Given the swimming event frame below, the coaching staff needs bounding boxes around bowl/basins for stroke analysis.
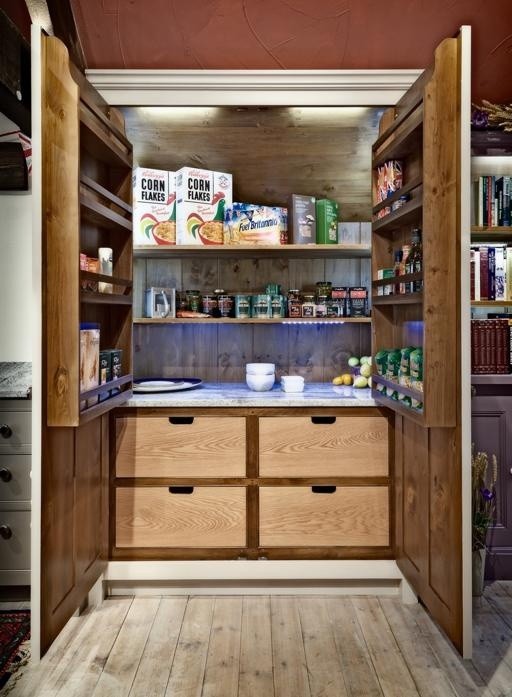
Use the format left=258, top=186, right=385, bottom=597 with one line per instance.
left=281, top=374, right=305, bottom=393
left=244, top=362, right=276, bottom=391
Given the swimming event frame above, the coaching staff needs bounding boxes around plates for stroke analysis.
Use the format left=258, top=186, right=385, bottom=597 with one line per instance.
left=132, top=376, right=204, bottom=393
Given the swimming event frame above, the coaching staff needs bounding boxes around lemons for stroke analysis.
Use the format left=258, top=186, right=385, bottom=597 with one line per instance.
left=334, top=375, right=352, bottom=386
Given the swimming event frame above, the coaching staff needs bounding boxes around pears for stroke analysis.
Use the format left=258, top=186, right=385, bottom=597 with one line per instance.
left=348, top=356, right=371, bottom=388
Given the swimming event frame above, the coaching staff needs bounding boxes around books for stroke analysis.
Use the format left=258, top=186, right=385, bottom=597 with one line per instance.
left=470, top=169, right=511, bottom=378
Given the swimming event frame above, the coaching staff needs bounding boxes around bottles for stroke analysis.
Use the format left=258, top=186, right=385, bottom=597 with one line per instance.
left=391, top=227, right=423, bottom=294
left=288, top=281, right=367, bottom=317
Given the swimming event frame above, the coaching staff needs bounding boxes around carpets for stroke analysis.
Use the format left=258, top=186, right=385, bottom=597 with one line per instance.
left=0, top=608, right=36, bottom=697
